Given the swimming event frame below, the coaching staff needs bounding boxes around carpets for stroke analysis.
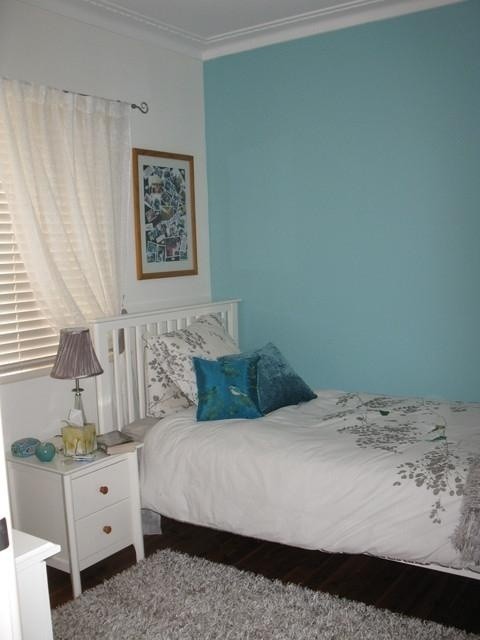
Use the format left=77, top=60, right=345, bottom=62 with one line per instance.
left=53, top=548, right=480, bottom=640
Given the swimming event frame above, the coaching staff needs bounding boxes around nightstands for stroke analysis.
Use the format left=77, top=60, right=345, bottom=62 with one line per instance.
left=13, top=441, right=145, bottom=598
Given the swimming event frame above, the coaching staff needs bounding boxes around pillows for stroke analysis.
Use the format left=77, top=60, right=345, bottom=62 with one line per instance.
left=137, top=314, right=316, bottom=425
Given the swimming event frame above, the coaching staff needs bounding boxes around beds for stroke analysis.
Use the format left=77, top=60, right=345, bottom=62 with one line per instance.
left=78, top=297, right=480, bottom=580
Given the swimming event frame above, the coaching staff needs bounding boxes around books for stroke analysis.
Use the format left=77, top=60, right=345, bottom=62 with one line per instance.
left=96, top=429, right=136, bottom=456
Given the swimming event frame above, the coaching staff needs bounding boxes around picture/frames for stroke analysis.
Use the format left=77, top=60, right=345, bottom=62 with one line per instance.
left=130, top=147, right=199, bottom=282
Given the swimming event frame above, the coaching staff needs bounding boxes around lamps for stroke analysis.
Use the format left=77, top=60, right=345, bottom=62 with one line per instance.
left=52, top=327, right=105, bottom=426
